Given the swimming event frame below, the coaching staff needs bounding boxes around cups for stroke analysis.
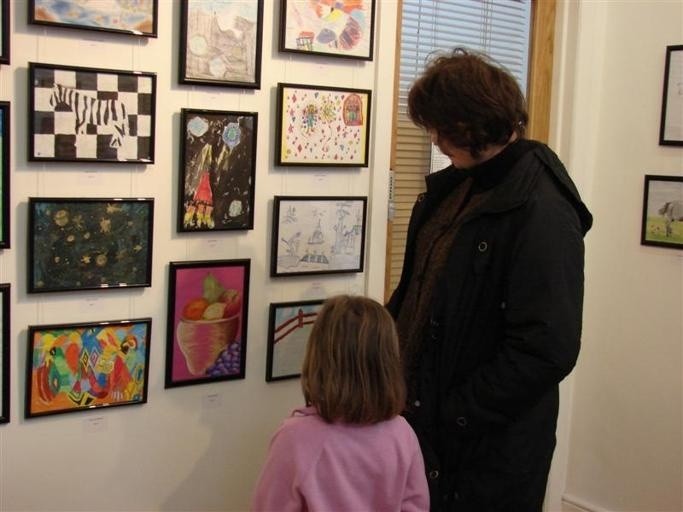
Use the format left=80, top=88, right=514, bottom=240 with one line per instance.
left=177, top=313, right=240, bottom=376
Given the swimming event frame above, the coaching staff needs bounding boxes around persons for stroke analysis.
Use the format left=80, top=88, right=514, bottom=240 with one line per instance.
left=384, top=46, right=593, bottom=512
left=247, top=294, right=432, bottom=512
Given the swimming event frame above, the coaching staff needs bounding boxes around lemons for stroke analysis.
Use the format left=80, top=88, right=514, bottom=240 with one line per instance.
left=201, top=303, right=226, bottom=320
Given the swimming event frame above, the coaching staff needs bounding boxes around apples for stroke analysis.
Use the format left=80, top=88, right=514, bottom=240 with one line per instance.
left=220, top=290, right=240, bottom=317
left=182, top=299, right=208, bottom=321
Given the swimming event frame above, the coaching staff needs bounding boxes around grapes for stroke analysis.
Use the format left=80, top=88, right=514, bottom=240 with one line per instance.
left=206, top=342, right=241, bottom=378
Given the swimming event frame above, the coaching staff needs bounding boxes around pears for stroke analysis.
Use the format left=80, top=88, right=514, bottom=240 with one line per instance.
left=202, top=272, right=220, bottom=302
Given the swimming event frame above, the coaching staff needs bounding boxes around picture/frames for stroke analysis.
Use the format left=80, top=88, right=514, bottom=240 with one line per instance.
left=638, top=44, right=682, bottom=250
